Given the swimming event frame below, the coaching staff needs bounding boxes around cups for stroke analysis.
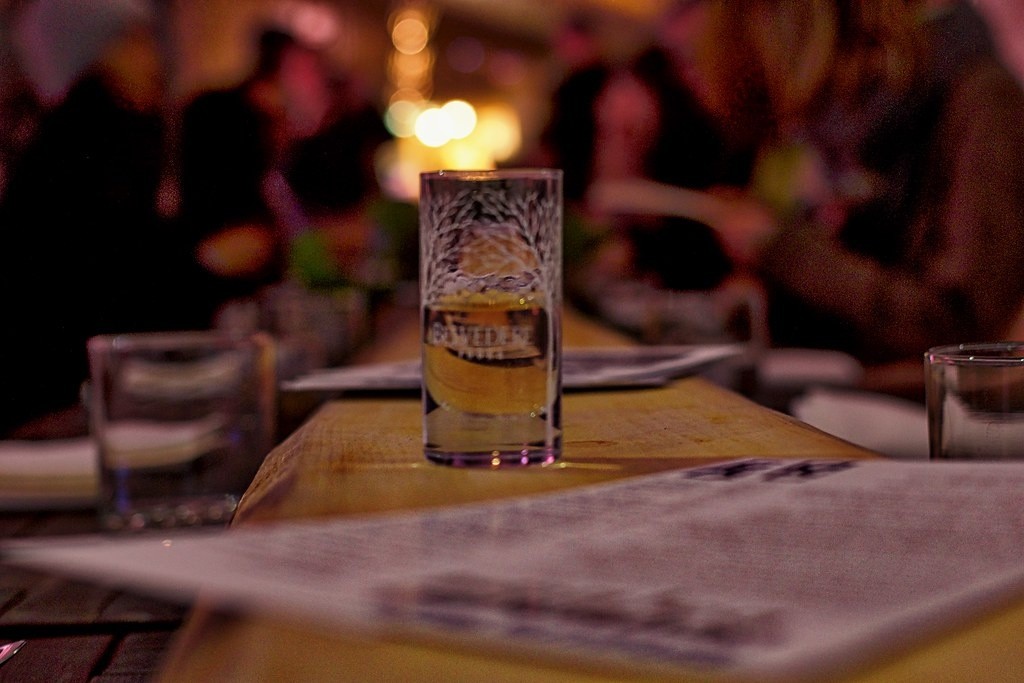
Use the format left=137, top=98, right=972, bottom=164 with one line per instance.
left=419, top=170, right=563, bottom=468
left=89, top=335, right=276, bottom=535
left=924, top=341, right=1024, bottom=461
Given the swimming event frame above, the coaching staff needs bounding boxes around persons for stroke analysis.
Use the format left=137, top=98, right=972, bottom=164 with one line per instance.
left=0, top=1, right=1024, bottom=402
